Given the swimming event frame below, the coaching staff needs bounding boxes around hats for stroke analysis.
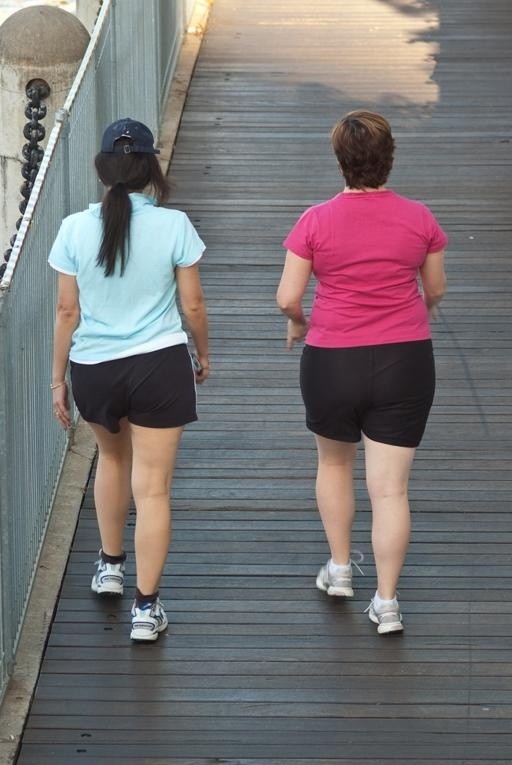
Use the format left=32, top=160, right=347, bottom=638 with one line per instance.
left=101, top=118, right=161, bottom=155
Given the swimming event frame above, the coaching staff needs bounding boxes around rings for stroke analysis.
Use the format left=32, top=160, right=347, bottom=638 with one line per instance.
left=56, top=413, right=59, bottom=416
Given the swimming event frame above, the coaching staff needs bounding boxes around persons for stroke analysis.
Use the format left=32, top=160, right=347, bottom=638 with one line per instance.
left=43, top=117, right=210, bottom=644
left=275, top=108, right=448, bottom=637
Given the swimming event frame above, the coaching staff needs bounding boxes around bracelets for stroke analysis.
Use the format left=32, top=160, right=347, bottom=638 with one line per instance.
left=50, top=380, right=66, bottom=390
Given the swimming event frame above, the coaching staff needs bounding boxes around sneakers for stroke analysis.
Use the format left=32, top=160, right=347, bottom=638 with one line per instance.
left=368, top=591, right=404, bottom=635
left=91, top=548, right=126, bottom=597
left=130, top=599, right=169, bottom=642
left=316, top=558, right=355, bottom=598
